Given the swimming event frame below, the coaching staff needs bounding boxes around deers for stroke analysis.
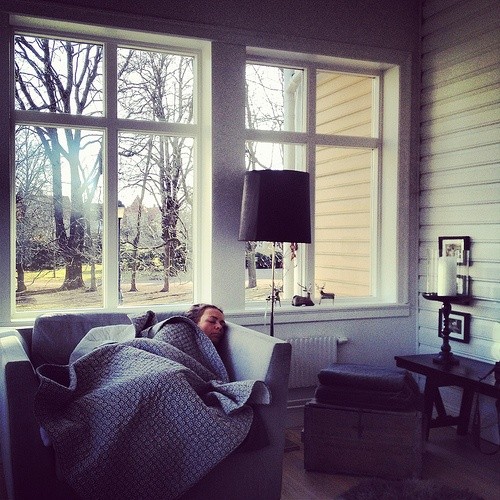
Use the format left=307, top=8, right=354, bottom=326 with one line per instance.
left=265, top=284, right=284, bottom=307
left=291, top=282, right=315, bottom=307
left=316, top=282, right=335, bottom=305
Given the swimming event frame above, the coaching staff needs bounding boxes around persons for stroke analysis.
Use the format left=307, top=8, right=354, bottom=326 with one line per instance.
left=186, top=306, right=226, bottom=347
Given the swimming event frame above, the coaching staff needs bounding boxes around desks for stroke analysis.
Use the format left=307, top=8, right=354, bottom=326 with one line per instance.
left=395, top=354, right=500, bottom=443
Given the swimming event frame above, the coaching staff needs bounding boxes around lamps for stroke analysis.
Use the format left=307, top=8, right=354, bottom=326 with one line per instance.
left=239, top=171, right=312, bottom=452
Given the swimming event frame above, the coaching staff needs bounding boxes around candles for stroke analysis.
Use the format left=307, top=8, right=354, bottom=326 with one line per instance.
left=438, top=257, right=457, bottom=296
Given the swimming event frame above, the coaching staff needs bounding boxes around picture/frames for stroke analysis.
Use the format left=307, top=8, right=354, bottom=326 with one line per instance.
left=439, top=236, right=470, bottom=265
left=455, top=275, right=470, bottom=297
left=438, top=309, right=471, bottom=342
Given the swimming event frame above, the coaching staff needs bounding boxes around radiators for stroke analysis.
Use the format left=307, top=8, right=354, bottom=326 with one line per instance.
left=284, top=335, right=337, bottom=388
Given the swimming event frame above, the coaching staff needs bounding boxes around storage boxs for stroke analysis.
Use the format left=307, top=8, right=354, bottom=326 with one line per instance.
left=302, top=394, right=425, bottom=481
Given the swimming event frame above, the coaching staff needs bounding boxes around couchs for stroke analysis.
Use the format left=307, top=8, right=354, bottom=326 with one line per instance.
left=0, top=311, right=292, bottom=500
